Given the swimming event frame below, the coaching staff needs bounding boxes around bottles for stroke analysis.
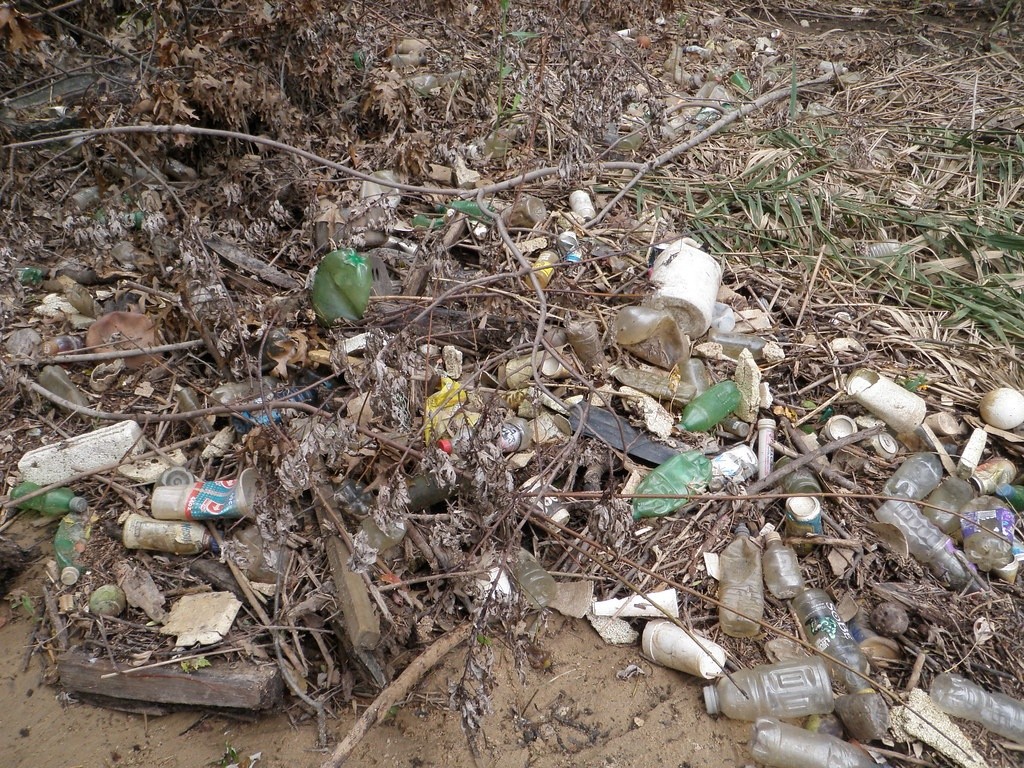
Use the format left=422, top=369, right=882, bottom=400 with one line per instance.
left=868, top=239, right=902, bottom=264
left=9, top=483, right=89, bottom=517
left=13, top=267, right=42, bottom=287
left=122, top=514, right=216, bottom=550
left=178, top=371, right=331, bottom=448
left=411, top=197, right=568, bottom=292
left=334, top=481, right=373, bottom=520
left=351, top=34, right=470, bottom=101
left=311, top=247, right=369, bottom=329
left=606, top=29, right=801, bottom=154
left=54, top=513, right=89, bottom=586
left=647, top=327, right=1024, bottom=767
left=817, top=61, right=850, bottom=74
left=63, top=150, right=203, bottom=327
left=806, top=102, right=841, bottom=123
left=41, top=335, right=84, bottom=355
left=357, top=323, right=607, bottom=610
left=40, top=367, right=89, bottom=416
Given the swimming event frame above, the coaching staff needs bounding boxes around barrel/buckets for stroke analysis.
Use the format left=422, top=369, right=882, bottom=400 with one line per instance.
left=639, top=245, right=723, bottom=341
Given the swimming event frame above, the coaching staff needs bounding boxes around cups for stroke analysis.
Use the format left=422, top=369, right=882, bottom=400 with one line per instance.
left=521, top=476, right=572, bottom=532
left=642, top=618, right=725, bottom=678
left=151, top=467, right=267, bottom=518
